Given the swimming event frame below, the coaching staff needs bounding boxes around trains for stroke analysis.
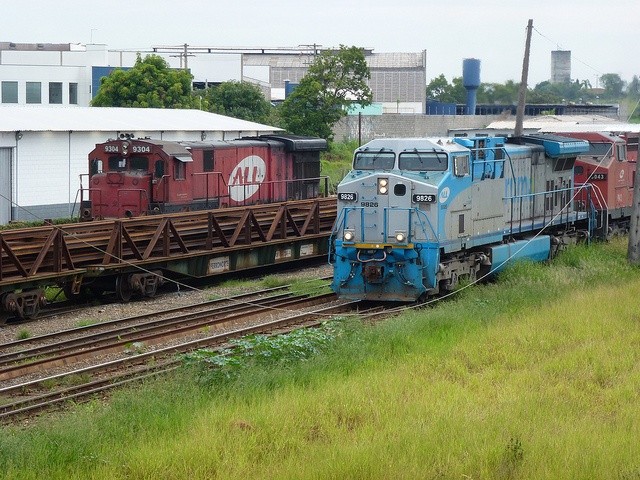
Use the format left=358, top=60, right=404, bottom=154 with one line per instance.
left=80, top=133, right=327, bottom=220
left=328, top=124, right=639, bottom=302
